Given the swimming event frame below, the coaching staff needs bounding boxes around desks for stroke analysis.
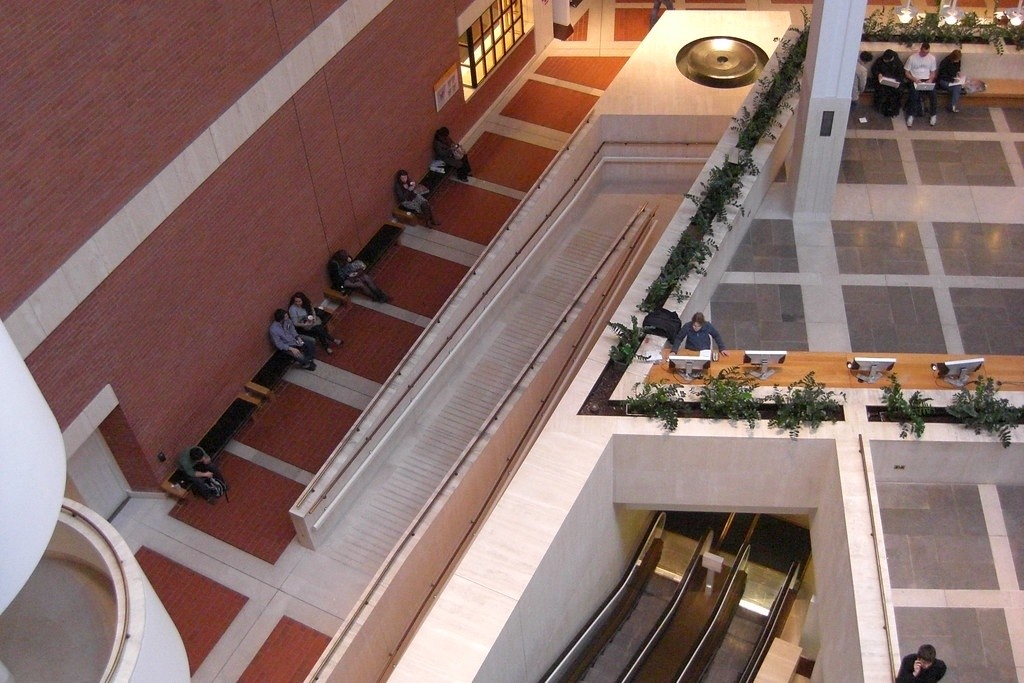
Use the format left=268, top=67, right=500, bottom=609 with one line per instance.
left=645, top=347, right=1024, bottom=390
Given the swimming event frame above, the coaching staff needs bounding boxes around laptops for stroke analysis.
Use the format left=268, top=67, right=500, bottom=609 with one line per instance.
left=879, top=76, right=900, bottom=89
left=913, top=82, right=935, bottom=91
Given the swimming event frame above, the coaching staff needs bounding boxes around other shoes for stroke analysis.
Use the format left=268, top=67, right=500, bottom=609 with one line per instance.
left=372, top=289, right=388, bottom=303
left=457, top=172, right=468, bottom=182
left=333, top=339, right=343, bottom=346
left=906, top=115, right=914, bottom=127
left=303, top=361, right=316, bottom=371
left=946, top=104, right=958, bottom=113
left=930, top=115, right=937, bottom=125
left=426, top=221, right=441, bottom=229
left=326, top=347, right=334, bottom=355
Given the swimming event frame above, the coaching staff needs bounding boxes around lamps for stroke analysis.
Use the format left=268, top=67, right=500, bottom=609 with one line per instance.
left=1005, top=0, right=1024, bottom=27
left=943, top=0, right=965, bottom=25
left=898, top=0, right=913, bottom=23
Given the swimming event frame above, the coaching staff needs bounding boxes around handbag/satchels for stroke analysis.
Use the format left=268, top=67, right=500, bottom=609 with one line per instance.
left=430, top=160, right=445, bottom=173
left=451, top=144, right=464, bottom=160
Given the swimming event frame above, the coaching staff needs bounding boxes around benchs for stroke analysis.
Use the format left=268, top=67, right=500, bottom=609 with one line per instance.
left=856, top=79, right=1024, bottom=108
left=322, top=220, right=405, bottom=309
left=245, top=305, right=333, bottom=398
left=161, top=392, right=260, bottom=502
left=393, top=154, right=460, bottom=226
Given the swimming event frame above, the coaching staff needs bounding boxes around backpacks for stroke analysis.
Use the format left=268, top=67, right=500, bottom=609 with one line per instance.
left=881, top=95, right=900, bottom=116
left=203, top=476, right=226, bottom=497
left=643, top=308, right=680, bottom=344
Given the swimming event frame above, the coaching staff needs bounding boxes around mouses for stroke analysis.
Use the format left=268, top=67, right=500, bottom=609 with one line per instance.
left=847, top=361, right=852, bottom=369
left=931, top=363, right=937, bottom=371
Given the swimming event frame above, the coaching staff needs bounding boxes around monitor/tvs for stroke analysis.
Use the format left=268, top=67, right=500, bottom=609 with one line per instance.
left=669, top=356, right=711, bottom=381
left=850, top=357, right=896, bottom=384
left=743, top=350, right=787, bottom=380
left=938, top=358, right=984, bottom=386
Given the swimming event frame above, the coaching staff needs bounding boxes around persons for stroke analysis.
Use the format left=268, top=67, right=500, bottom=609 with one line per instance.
left=395, top=170, right=441, bottom=228
left=328, top=250, right=390, bottom=302
left=871, top=50, right=905, bottom=111
left=937, top=50, right=961, bottom=112
left=651, top=0, right=673, bottom=26
left=433, top=127, right=470, bottom=181
left=268, top=309, right=316, bottom=371
left=668, top=312, right=729, bottom=357
left=904, top=43, right=937, bottom=127
left=175, top=446, right=230, bottom=505
left=896, top=645, right=946, bottom=683
left=288, top=292, right=343, bottom=355
left=850, top=52, right=872, bottom=108
